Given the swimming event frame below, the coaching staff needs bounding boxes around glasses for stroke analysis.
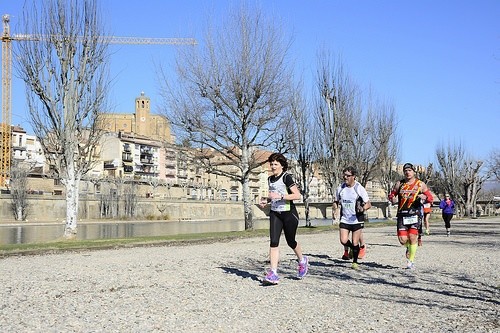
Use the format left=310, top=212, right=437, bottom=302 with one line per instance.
left=343, top=174, right=353, bottom=178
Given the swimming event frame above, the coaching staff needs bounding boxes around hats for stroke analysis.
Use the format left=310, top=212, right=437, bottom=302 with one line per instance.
left=403, top=163, right=414, bottom=171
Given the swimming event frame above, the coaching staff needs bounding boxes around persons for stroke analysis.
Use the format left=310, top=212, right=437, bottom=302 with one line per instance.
left=386, top=162, right=434, bottom=268
left=332, top=165, right=372, bottom=270
left=423, top=201, right=433, bottom=235
left=260, top=152, right=308, bottom=285
left=342, top=228, right=366, bottom=259
left=439, top=192, right=456, bottom=237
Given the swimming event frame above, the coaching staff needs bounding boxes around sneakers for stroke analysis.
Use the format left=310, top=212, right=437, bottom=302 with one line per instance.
left=359, top=245, right=366, bottom=258
left=298, top=257, right=307, bottom=278
left=264, top=271, right=279, bottom=284
left=352, top=263, right=358, bottom=270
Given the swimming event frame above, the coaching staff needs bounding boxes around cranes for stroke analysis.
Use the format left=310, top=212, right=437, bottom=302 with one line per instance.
left=0, top=14, right=200, bottom=194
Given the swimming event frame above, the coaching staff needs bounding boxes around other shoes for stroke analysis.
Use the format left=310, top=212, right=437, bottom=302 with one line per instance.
left=418, top=239, right=421, bottom=246
left=407, top=260, right=414, bottom=268
left=425, top=229, right=430, bottom=234
left=405, top=248, right=410, bottom=259
left=342, top=252, right=352, bottom=259
left=447, top=230, right=451, bottom=236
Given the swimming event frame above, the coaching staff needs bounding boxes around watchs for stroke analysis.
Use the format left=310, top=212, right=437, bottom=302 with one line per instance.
left=281, top=193, right=284, bottom=200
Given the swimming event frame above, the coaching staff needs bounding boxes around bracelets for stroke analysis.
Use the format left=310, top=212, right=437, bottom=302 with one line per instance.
left=363, top=207, right=365, bottom=211
